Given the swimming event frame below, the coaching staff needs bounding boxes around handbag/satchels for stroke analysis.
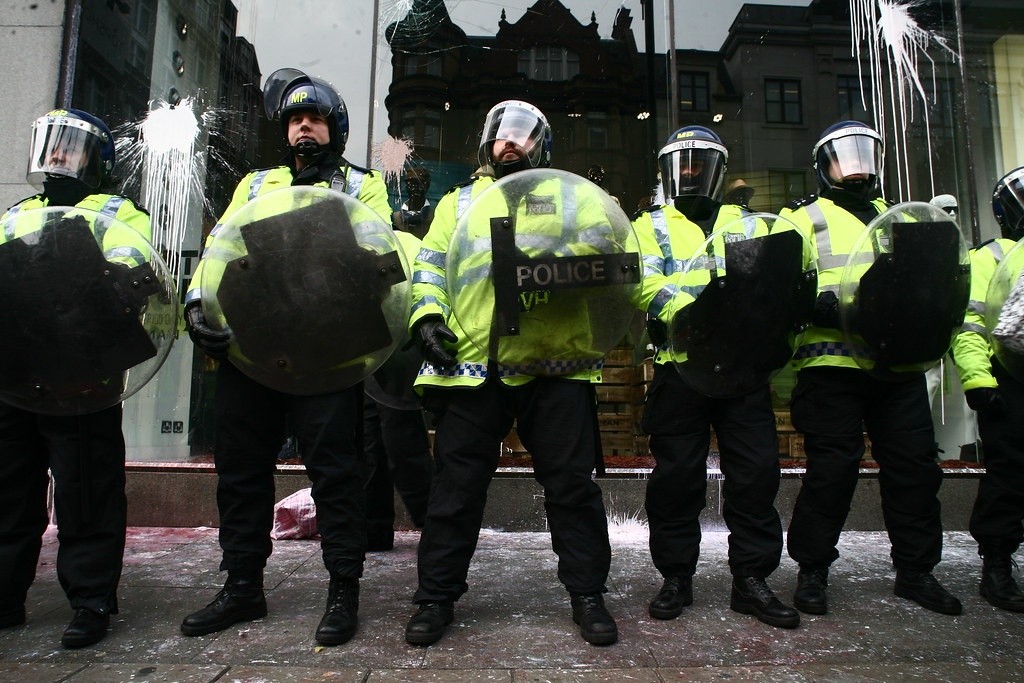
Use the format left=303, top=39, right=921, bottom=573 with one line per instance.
left=270, top=488, right=318, bottom=540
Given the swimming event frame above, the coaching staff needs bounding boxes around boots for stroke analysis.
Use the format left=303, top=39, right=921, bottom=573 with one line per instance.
left=980, top=550, right=1024, bottom=611
left=180, top=568, right=267, bottom=634
left=316, top=576, right=359, bottom=644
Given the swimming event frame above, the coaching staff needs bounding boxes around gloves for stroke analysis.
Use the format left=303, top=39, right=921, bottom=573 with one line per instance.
left=187, top=305, right=230, bottom=360
left=671, top=302, right=696, bottom=352
left=401, top=314, right=458, bottom=368
left=966, top=388, right=1009, bottom=414
left=812, top=291, right=839, bottom=327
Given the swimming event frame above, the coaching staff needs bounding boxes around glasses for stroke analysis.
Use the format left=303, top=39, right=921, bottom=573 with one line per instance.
left=941, top=206, right=959, bottom=215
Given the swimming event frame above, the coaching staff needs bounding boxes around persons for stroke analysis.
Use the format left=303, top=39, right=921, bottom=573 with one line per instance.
left=727, top=176, right=758, bottom=214
left=947, top=166, right=1024, bottom=614
left=390, top=230, right=433, bottom=531
left=1, top=106, right=151, bottom=651
left=182, top=67, right=395, bottom=646
left=390, top=166, right=435, bottom=240
left=406, top=98, right=620, bottom=646
left=623, top=125, right=800, bottom=630
left=768, top=120, right=964, bottom=616
left=589, top=162, right=608, bottom=188
left=922, top=193, right=983, bottom=462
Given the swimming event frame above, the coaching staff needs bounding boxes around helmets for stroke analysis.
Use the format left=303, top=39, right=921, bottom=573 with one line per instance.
left=816, top=121, right=883, bottom=196
left=668, top=126, right=723, bottom=193
left=277, top=76, right=351, bottom=154
left=487, top=105, right=553, bottom=170
left=45, top=109, right=115, bottom=175
left=992, top=166, right=1024, bottom=228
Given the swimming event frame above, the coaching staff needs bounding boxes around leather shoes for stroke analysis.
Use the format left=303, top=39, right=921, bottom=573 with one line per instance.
left=0, top=611, right=25, bottom=628
left=63, top=607, right=111, bottom=644
left=731, top=575, right=801, bottom=626
left=895, top=568, right=962, bottom=613
left=649, top=575, right=695, bottom=619
left=794, top=571, right=828, bottom=615
left=406, top=600, right=455, bottom=643
left=571, top=593, right=618, bottom=644
